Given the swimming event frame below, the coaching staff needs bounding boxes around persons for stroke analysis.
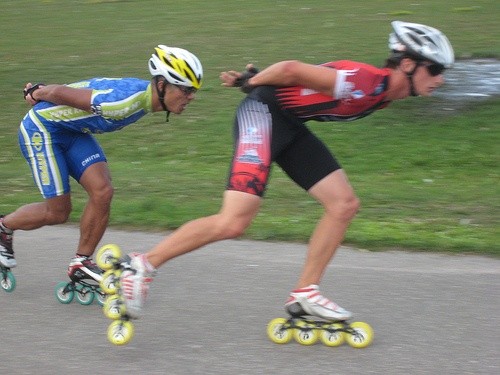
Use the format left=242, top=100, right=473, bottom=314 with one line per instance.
left=95, top=20, right=456, bottom=348
left=0, top=44, right=203, bottom=306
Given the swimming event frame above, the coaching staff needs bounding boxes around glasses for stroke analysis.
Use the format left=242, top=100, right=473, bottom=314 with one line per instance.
left=414, top=60, right=444, bottom=76
left=174, top=84, right=198, bottom=95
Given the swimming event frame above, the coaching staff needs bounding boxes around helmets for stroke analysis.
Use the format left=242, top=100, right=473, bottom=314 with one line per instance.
left=148, top=45, right=203, bottom=89
left=388, top=21, right=455, bottom=68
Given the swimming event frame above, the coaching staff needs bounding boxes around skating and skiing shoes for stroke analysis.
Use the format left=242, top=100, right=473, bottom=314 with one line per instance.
left=96, top=243, right=157, bottom=345
left=54, top=255, right=108, bottom=306
left=0, top=215, right=17, bottom=292
left=267, top=284, right=374, bottom=348
